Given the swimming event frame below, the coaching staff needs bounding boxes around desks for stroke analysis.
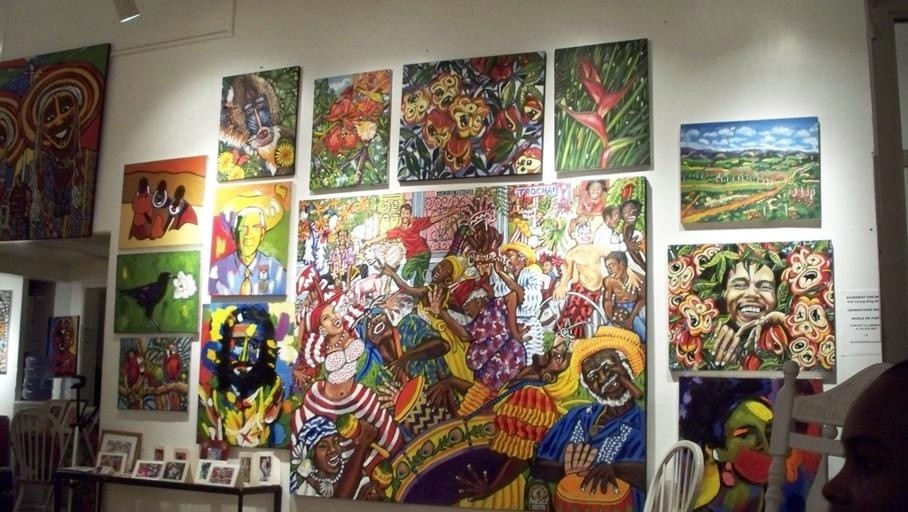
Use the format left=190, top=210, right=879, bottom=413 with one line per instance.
left=49, top=466, right=284, bottom=512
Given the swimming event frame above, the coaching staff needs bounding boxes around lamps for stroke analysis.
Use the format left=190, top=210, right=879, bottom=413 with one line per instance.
left=112, top=0, right=143, bottom=24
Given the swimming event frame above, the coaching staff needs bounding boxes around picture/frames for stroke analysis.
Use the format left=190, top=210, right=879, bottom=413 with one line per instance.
left=44, top=397, right=101, bottom=432
left=88, top=424, right=287, bottom=492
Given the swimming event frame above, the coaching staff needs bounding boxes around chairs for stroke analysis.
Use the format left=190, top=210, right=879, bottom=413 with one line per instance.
left=760, top=355, right=901, bottom=512
left=642, top=435, right=708, bottom=512
left=7, top=406, right=76, bottom=511
